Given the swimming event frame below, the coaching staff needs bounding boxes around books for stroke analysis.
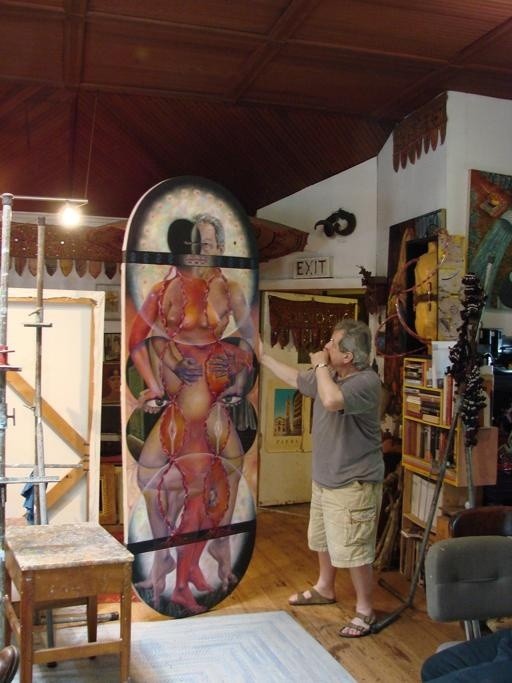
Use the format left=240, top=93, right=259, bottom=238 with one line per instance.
left=400, top=357, right=500, bottom=589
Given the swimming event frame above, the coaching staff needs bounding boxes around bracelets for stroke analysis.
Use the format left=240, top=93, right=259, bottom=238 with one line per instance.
left=314, top=363, right=329, bottom=372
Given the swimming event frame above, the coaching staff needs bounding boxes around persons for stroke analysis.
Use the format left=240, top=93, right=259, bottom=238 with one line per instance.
left=259, top=317, right=386, bottom=640
left=421, top=627, right=512, bottom=682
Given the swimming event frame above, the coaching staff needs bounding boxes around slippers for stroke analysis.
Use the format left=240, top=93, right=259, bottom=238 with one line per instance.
left=339, top=611, right=377, bottom=637
left=288, top=587, right=337, bottom=606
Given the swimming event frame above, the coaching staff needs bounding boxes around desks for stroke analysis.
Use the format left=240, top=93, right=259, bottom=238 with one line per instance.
left=1, top=518, right=138, bottom=683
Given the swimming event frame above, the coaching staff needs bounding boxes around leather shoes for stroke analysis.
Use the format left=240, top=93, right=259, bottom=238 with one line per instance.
left=0, top=645, right=20, bottom=683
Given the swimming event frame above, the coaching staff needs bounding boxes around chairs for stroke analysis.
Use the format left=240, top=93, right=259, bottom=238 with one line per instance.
left=448, top=504, right=511, bottom=640
left=421, top=535, right=511, bottom=682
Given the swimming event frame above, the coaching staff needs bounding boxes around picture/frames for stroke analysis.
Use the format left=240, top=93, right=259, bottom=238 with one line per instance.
left=102, top=361, right=121, bottom=404
left=95, top=281, right=121, bottom=323
left=103, top=331, right=121, bottom=362
left=98, top=463, right=118, bottom=525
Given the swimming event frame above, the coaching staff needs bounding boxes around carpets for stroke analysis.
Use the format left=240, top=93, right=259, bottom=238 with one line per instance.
left=9, top=609, right=359, bottom=683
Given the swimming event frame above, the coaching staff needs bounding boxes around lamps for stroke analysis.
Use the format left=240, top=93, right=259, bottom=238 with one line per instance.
left=53, top=86, right=105, bottom=229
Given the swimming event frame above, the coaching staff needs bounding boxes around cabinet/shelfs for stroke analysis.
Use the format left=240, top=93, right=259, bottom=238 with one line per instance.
left=398, top=354, right=499, bottom=595
